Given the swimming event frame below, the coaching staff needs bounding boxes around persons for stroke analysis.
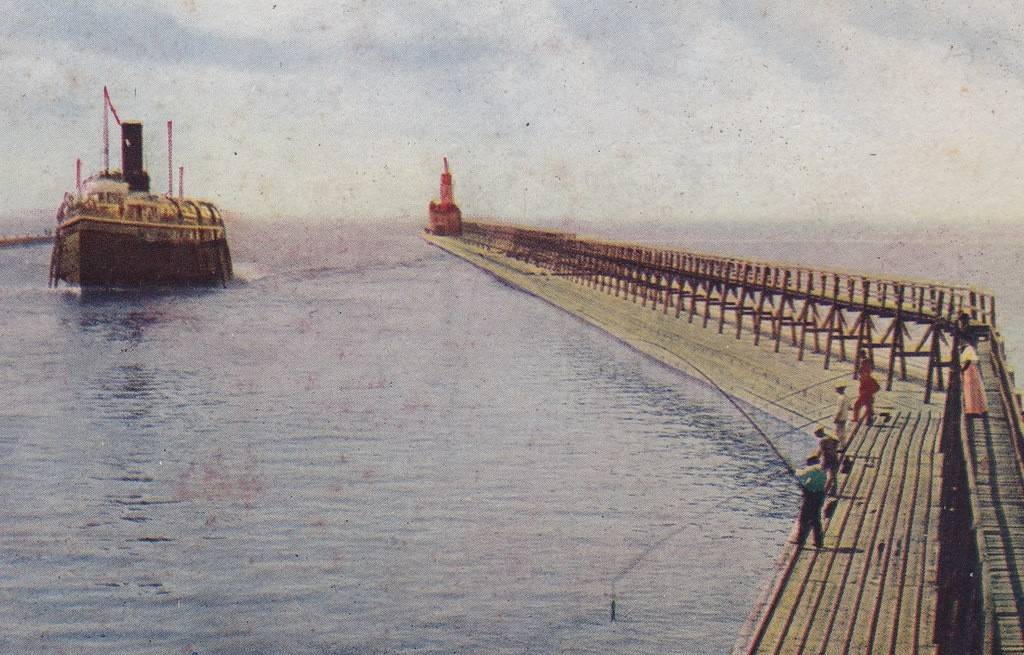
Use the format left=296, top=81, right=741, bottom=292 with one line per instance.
left=958, top=312, right=978, bottom=348
left=858, top=350, right=871, bottom=375
left=813, top=426, right=840, bottom=496
left=834, top=381, right=848, bottom=452
left=850, top=367, right=874, bottom=426
left=958, top=333, right=988, bottom=417
left=789, top=454, right=826, bottom=548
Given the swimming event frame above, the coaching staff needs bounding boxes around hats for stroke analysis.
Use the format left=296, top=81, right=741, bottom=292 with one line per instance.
left=835, top=383, right=847, bottom=389
left=958, top=313, right=970, bottom=321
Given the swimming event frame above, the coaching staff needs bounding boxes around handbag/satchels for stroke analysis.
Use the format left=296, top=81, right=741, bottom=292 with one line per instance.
left=870, top=378, right=880, bottom=396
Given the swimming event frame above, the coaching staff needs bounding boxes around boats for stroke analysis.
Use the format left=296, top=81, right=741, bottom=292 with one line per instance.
left=48, top=86, right=235, bottom=292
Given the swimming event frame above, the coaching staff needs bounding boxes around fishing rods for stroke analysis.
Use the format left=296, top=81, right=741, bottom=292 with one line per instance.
left=610, top=470, right=796, bottom=622
left=749, top=369, right=859, bottom=412
left=706, top=414, right=835, bottom=471
